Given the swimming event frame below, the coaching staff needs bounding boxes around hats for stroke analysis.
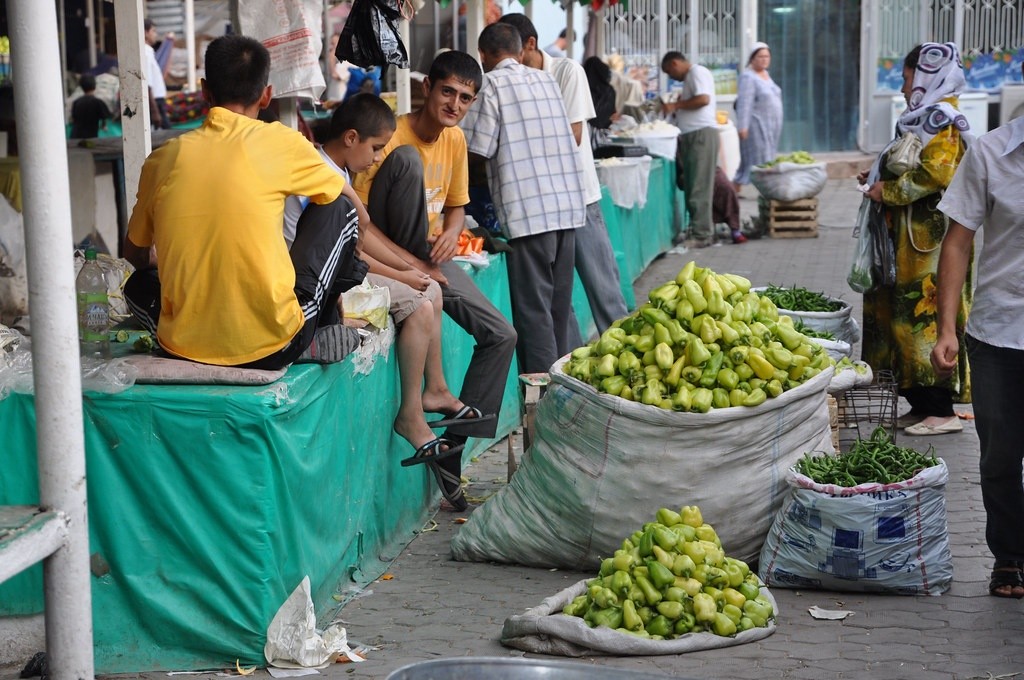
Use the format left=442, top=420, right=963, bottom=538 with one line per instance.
left=744, top=42, right=769, bottom=65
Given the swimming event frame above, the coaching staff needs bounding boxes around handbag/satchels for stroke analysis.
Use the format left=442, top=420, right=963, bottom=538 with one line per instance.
left=335, top=0, right=410, bottom=70
left=885, top=132, right=923, bottom=175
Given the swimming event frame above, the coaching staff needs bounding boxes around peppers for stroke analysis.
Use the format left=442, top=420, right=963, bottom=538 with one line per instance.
left=758, top=151, right=816, bottom=167
left=794, top=426, right=940, bottom=488
left=562, top=506, right=777, bottom=642
left=561, top=259, right=869, bottom=412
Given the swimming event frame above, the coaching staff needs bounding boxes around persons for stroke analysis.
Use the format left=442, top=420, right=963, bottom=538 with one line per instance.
left=283, top=90, right=496, bottom=468
left=123, top=35, right=370, bottom=370
left=455, top=22, right=586, bottom=416
left=145, top=17, right=171, bottom=131
left=542, top=26, right=576, bottom=58
left=69, top=72, right=109, bottom=139
left=731, top=41, right=783, bottom=193
left=814, top=13, right=846, bottom=122
left=495, top=12, right=628, bottom=353
left=349, top=51, right=517, bottom=511
left=679, top=163, right=747, bottom=244
left=662, top=51, right=721, bottom=250
left=855, top=42, right=973, bottom=434
left=929, top=116, right=1024, bottom=596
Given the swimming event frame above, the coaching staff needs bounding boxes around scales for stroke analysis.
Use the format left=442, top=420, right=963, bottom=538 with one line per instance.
left=594, top=142, right=649, bottom=158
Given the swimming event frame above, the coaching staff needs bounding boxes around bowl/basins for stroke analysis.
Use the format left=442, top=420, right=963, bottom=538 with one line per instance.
left=608, top=135, right=634, bottom=144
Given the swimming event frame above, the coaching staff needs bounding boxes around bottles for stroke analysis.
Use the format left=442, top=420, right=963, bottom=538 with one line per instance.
left=74, top=249, right=109, bottom=358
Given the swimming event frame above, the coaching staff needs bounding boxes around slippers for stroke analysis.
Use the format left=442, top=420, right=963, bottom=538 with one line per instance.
left=426, top=405, right=497, bottom=426
left=401, top=438, right=466, bottom=467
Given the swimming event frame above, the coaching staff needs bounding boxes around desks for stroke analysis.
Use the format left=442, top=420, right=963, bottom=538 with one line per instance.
left=0, top=149, right=693, bottom=667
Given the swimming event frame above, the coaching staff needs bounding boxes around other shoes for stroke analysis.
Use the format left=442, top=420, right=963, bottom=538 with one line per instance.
left=683, top=238, right=713, bottom=247
left=295, top=324, right=361, bottom=364
left=905, top=415, right=963, bottom=434
left=436, top=449, right=468, bottom=510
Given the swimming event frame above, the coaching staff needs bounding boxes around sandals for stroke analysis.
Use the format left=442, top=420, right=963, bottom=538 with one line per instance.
left=988, top=559, right=1024, bottom=598
